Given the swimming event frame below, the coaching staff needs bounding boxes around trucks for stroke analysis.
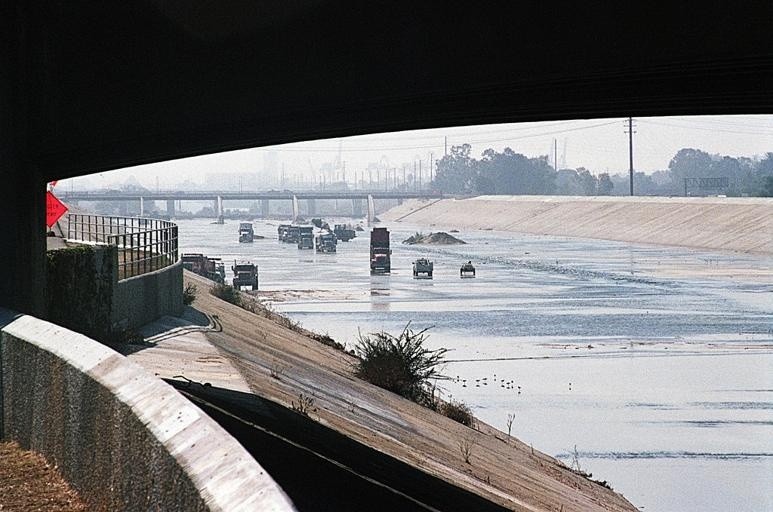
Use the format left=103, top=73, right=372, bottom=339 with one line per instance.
left=180, top=253, right=225, bottom=284
left=334, top=224, right=355, bottom=242
left=315, top=234, right=336, bottom=252
left=277, top=225, right=314, bottom=250
left=231, top=259, right=258, bottom=290
left=370, top=228, right=392, bottom=273
left=238, top=223, right=254, bottom=243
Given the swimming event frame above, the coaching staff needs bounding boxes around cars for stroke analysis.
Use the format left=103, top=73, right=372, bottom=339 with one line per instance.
left=412, top=260, right=433, bottom=276
left=460, top=260, right=475, bottom=276
